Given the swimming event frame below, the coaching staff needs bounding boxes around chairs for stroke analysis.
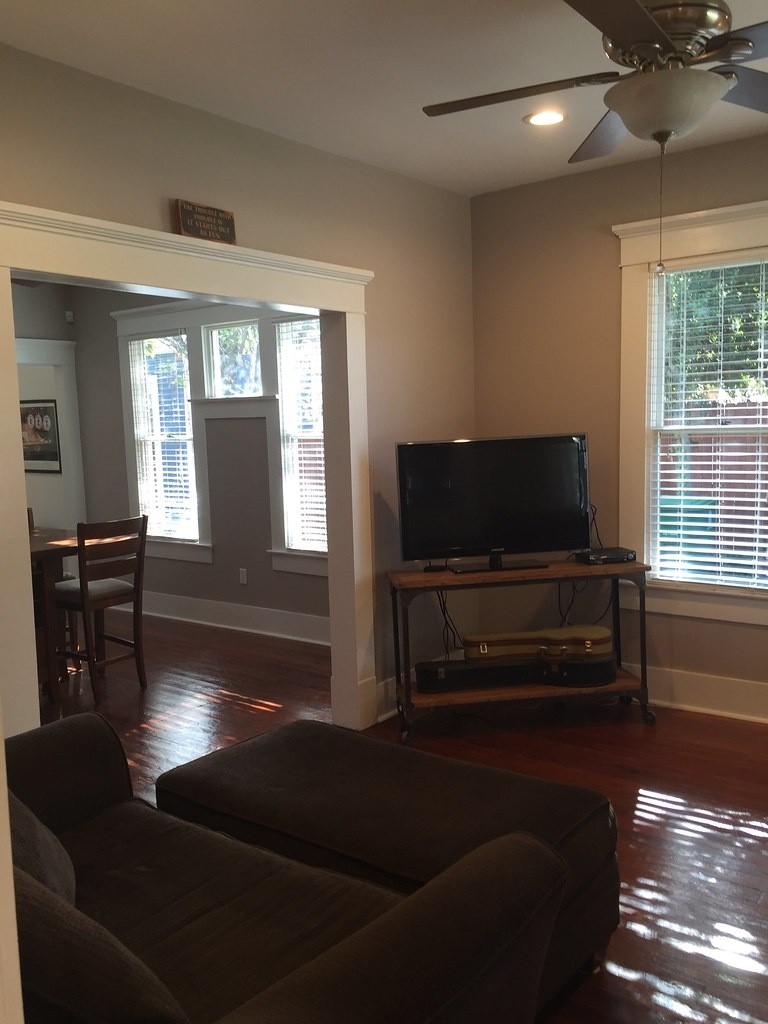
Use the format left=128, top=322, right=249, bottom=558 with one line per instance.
left=55, top=515, right=148, bottom=706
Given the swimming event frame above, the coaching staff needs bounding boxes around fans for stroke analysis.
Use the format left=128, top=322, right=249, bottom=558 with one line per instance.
left=423, top=0, right=768, bottom=276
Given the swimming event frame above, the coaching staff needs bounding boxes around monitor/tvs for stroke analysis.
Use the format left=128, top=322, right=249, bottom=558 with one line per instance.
left=396, top=431, right=592, bottom=575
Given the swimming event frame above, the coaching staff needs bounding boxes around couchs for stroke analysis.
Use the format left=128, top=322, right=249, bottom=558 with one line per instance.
left=4, top=710, right=568, bottom=1024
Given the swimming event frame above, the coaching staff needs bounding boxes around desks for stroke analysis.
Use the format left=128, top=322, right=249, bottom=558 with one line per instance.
left=385, top=557, right=657, bottom=740
left=29, top=527, right=79, bottom=704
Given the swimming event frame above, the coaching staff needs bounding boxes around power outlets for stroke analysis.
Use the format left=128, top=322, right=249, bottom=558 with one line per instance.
left=239, top=568, right=248, bottom=585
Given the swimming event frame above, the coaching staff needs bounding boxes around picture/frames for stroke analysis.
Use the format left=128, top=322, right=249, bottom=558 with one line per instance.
left=20, top=399, right=63, bottom=475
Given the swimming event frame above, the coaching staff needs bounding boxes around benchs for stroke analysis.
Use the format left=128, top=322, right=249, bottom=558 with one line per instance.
left=154, top=719, right=621, bottom=893
